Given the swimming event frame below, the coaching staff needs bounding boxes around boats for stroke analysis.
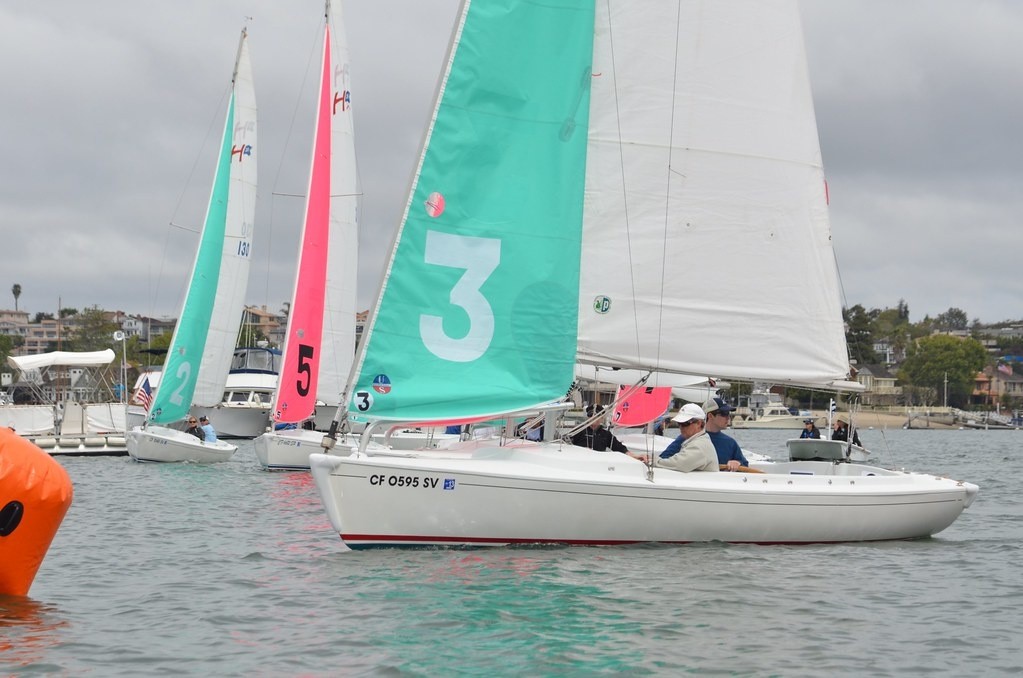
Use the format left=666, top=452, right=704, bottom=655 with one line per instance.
left=1, top=296, right=129, bottom=459
left=188, top=344, right=284, bottom=441
left=785, top=397, right=872, bottom=464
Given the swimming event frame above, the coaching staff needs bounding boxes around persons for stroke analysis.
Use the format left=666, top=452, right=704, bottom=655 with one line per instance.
left=185, top=415, right=216, bottom=443
left=53, top=401, right=64, bottom=435
left=659, top=398, right=748, bottom=472
left=111, top=380, right=124, bottom=402
left=642, top=403, right=719, bottom=473
left=800, top=419, right=821, bottom=439
left=514, top=417, right=545, bottom=442
left=832, top=416, right=862, bottom=447
left=569, top=404, right=648, bottom=463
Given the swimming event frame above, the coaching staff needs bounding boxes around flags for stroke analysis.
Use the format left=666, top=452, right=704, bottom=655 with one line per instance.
left=133, top=376, right=153, bottom=411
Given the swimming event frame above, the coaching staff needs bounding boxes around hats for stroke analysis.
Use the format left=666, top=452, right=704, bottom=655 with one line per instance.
left=837, top=415, right=848, bottom=424
left=200, top=416, right=210, bottom=420
left=671, top=403, right=705, bottom=423
left=586, top=405, right=605, bottom=417
left=804, top=418, right=814, bottom=424
left=702, top=398, right=737, bottom=418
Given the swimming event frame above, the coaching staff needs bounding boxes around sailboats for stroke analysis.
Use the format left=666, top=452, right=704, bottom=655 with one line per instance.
left=250, top=0, right=574, bottom=474
left=306, top=0, right=980, bottom=556
left=574, top=362, right=774, bottom=468
left=121, top=11, right=260, bottom=466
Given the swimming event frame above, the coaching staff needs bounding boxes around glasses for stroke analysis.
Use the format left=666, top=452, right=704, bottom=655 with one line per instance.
left=678, top=420, right=698, bottom=426
left=189, top=421, right=197, bottom=424
left=716, top=412, right=731, bottom=418
left=201, top=419, right=207, bottom=422
